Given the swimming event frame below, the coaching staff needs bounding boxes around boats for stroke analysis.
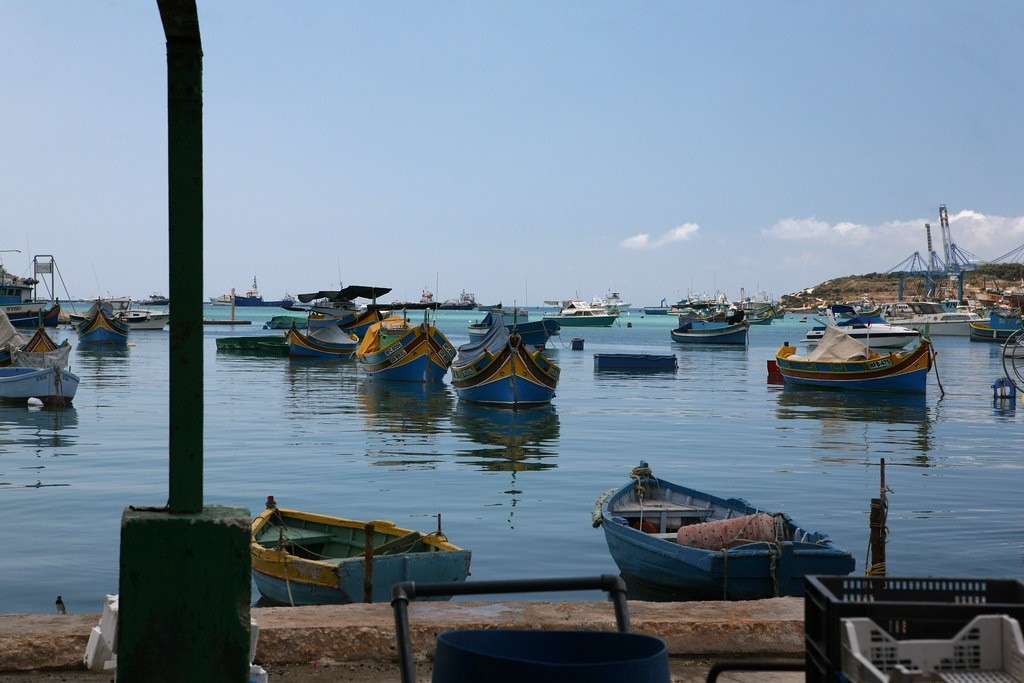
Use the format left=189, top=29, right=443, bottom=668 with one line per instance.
left=75, top=296, right=131, bottom=345
left=367, top=286, right=502, bottom=311
left=644, top=288, right=786, bottom=345
left=969, top=311, right=1024, bottom=359
left=69, top=295, right=169, bottom=331
left=449, top=308, right=561, bottom=405
left=1, top=308, right=72, bottom=369
left=356, top=307, right=457, bottom=383
left=467, top=309, right=561, bottom=352
left=543, top=289, right=633, bottom=327
left=0, top=365, right=81, bottom=407
left=593, top=461, right=856, bottom=604
left=800, top=288, right=1024, bottom=348
left=209, top=275, right=297, bottom=307
left=44, top=297, right=61, bottom=327
left=265, top=285, right=391, bottom=343
left=0, top=249, right=48, bottom=328
left=285, top=317, right=359, bottom=359
left=776, top=318, right=933, bottom=395
left=248, top=496, right=473, bottom=606
